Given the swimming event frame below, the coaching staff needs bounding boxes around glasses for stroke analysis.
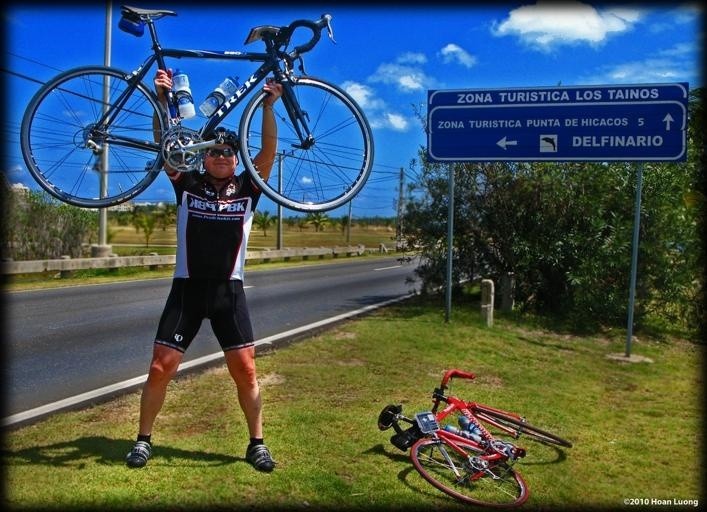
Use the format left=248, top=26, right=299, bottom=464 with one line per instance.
left=206, top=147, right=234, bottom=158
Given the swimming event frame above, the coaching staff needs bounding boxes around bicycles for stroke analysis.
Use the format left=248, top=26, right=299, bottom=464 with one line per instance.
left=375, top=363, right=574, bottom=510
left=17, top=2, right=378, bottom=214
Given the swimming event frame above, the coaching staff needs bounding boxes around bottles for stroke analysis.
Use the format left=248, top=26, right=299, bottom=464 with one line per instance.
left=457, top=415, right=483, bottom=438
left=441, top=424, right=482, bottom=443
left=198, top=72, right=240, bottom=120
left=171, top=67, right=196, bottom=120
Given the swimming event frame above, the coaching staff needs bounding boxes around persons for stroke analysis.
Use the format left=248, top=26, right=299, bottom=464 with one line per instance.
left=126, top=69, right=283, bottom=475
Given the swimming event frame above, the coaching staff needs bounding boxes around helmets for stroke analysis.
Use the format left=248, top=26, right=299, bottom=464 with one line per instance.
left=204, top=126, right=239, bottom=155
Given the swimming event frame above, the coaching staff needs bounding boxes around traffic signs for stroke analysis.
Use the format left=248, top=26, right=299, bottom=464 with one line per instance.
left=423, top=79, right=690, bottom=165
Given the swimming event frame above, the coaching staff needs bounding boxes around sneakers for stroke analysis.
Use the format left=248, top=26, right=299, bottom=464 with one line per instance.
left=126, top=441, right=152, bottom=467
left=246, top=444, right=278, bottom=471
